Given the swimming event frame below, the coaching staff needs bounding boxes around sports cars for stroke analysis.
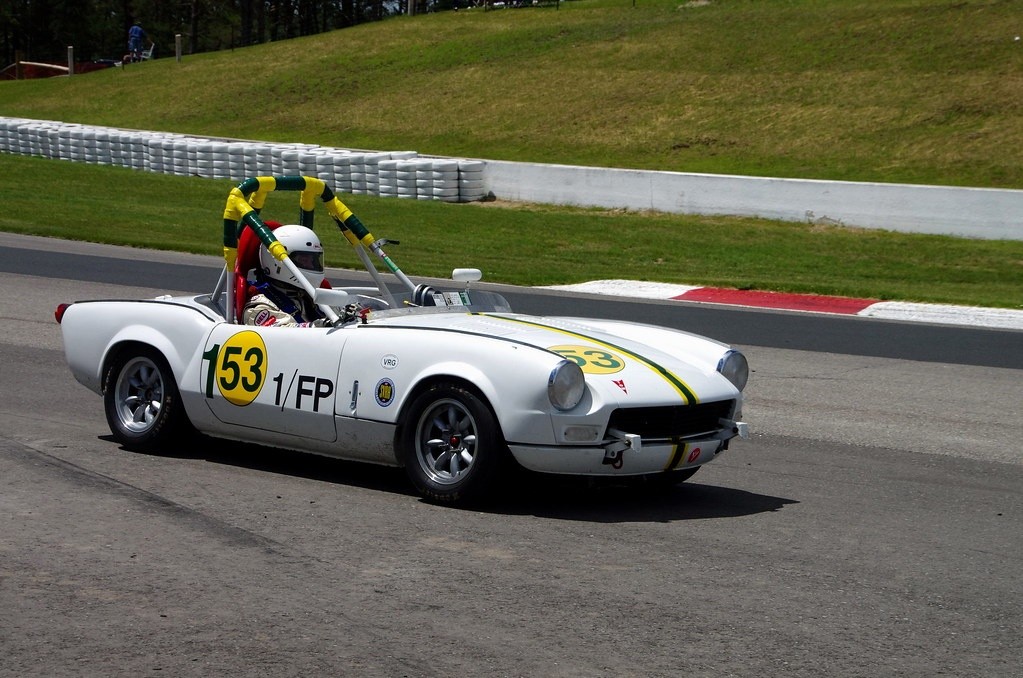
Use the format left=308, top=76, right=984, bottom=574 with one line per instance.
left=53, top=177, right=750, bottom=507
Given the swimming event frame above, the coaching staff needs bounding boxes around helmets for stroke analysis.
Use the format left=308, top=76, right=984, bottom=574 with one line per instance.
left=258, top=224, right=324, bottom=289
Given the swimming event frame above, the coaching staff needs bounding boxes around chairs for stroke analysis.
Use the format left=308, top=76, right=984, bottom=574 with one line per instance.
left=235, top=221, right=332, bottom=324
left=139, top=43, right=155, bottom=63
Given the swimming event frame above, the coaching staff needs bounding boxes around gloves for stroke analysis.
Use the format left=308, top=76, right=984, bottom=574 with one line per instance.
left=323, top=317, right=332, bottom=327
left=338, top=303, right=365, bottom=323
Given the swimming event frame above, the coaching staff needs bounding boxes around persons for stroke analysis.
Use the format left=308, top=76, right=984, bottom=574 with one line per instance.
left=242, top=224, right=368, bottom=327
left=127, top=20, right=151, bottom=63
